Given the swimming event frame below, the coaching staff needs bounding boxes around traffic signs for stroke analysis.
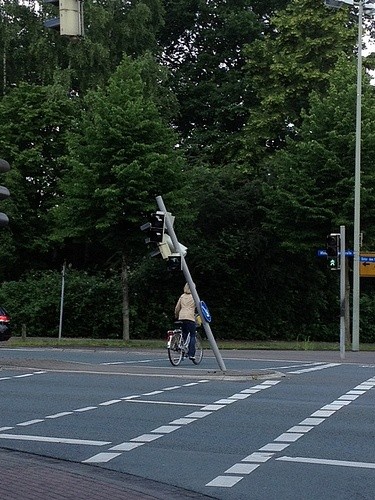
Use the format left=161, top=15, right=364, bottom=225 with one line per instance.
left=359, top=250, right=375, bottom=277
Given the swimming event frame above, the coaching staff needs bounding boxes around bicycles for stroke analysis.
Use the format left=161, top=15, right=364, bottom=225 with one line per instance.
left=167, top=314, right=203, bottom=367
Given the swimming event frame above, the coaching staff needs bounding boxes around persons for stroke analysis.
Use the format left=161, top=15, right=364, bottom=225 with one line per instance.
left=175, top=284, right=196, bottom=360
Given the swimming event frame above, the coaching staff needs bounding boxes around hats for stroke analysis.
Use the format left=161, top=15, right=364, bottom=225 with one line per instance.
left=184, top=281, right=195, bottom=293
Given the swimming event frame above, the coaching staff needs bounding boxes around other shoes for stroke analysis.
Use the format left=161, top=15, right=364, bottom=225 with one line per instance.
left=189, top=356, right=196, bottom=360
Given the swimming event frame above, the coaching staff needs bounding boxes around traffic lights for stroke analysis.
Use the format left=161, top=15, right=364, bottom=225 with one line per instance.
left=147, top=210, right=166, bottom=242
left=326, top=233, right=340, bottom=273
left=140, top=222, right=172, bottom=262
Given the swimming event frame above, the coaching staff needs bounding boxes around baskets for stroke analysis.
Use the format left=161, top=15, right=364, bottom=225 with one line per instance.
left=194, top=314, right=201, bottom=327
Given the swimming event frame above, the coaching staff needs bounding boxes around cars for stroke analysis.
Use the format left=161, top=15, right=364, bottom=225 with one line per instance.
left=0, top=305, right=14, bottom=341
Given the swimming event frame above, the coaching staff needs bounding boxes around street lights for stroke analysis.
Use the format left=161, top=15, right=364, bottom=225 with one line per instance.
left=322, top=0, right=374, bottom=351
